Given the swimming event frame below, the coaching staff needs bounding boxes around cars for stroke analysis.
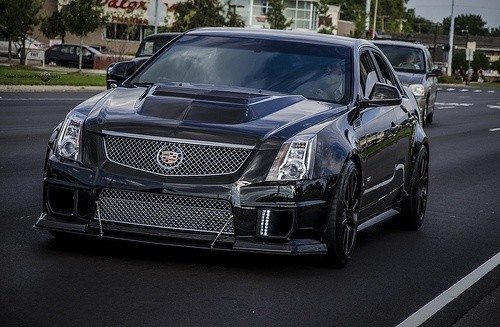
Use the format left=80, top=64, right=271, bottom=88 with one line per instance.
left=33, top=27, right=431, bottom=271
left=0, top=36, right=42, bottom=59
left=106, top=32, right=185, bottom=94
left=367, top=39, right=443, bottom=130
left=41, top=43, right=102, bottom=70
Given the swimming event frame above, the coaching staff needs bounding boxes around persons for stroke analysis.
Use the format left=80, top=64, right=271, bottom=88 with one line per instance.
left=398, top=54, right=420, bottom=69
left=437, top=64, right=483, bottom=82
left=305, top=59, right=345, bottom=102
